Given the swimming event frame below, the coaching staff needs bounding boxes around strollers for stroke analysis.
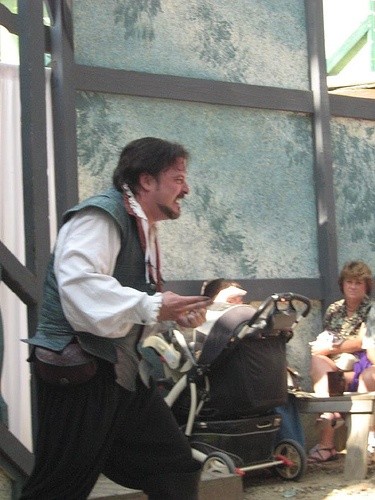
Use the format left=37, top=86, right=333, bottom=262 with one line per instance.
left=158, top=292, right=312, bottom=481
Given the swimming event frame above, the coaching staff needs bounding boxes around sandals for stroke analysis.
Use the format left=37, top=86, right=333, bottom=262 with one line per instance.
left=317, top=411, right=341, bottom=423
left=306, top=446, right=338, bottom=463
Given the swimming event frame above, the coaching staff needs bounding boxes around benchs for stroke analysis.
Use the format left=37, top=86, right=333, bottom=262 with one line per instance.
left=299, top=393, right=375, bottom=481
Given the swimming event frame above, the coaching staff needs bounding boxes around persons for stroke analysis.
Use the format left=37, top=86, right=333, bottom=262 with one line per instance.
left=305, top=259, right=375, bottom=463
left=189, top=278, right=254, bottom=362
left=20, top=137, right=213, bottom=500
